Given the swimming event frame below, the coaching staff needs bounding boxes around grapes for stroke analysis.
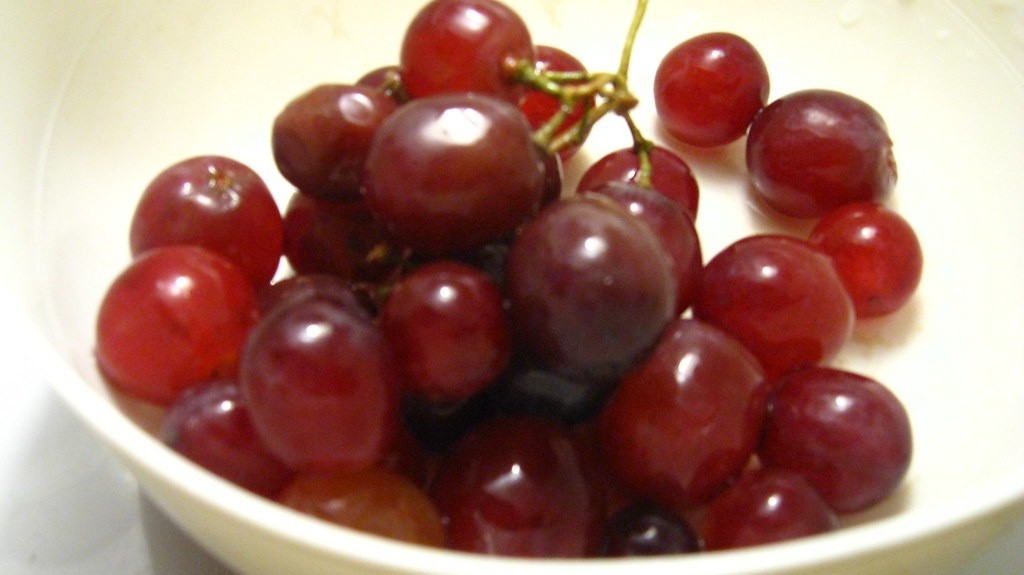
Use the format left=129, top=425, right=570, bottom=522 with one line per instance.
left=94, top=0, right=922, bottom=557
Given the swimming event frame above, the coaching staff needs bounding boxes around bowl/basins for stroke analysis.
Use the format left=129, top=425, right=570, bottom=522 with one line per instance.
left=26, top=0, right=1023, bottom=575
